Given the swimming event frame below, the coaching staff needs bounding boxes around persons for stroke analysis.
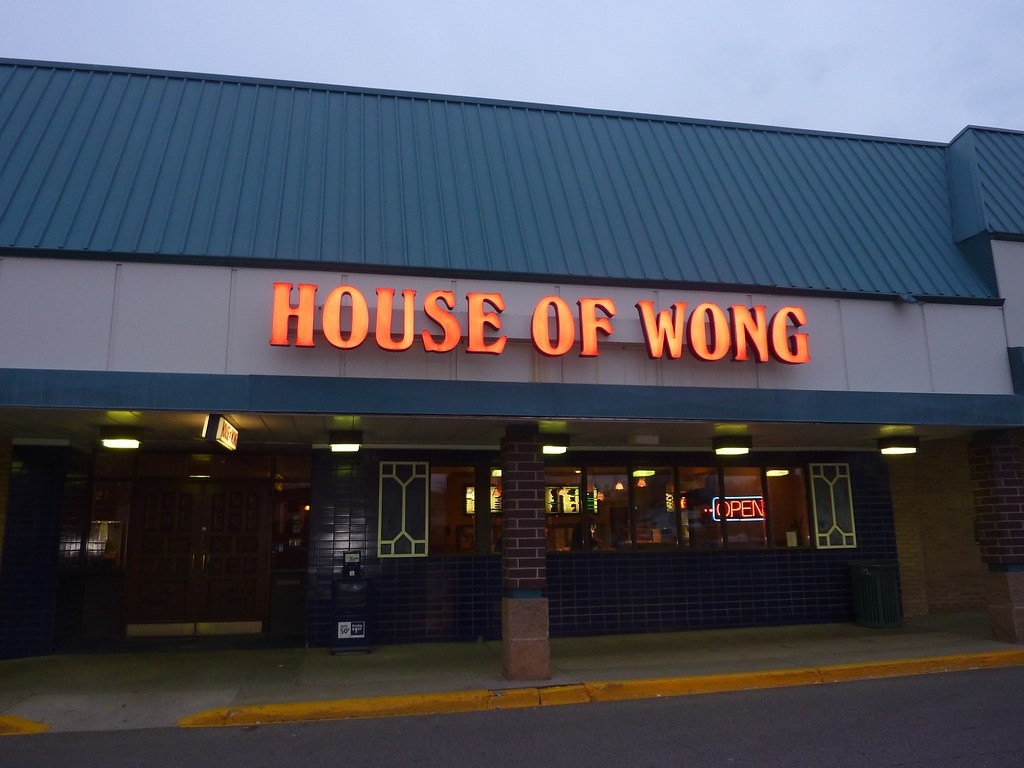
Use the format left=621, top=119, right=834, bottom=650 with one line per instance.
left=569, top=516, right=604, bottom=553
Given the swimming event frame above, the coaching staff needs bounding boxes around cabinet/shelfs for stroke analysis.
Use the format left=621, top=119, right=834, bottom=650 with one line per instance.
left=628, top=528, right=662, bottom=544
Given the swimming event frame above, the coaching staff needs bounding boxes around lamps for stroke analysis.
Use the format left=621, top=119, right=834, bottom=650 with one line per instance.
left=597, top=491, right=605, bottom=502
left=766, top=470, right=789, bottom=477
left=100, top=432, right=143, bottom=448
left=541, top=444, right=569, bottom=455
left=636, top=478, right=646, bottom=487
left=877, top=436, right=918, bottom=455
left=711, top=436, right=753, bottom=456
left=613, top=474, right=624, bottom=490
left=328, top=443, right=363, bottom=452
